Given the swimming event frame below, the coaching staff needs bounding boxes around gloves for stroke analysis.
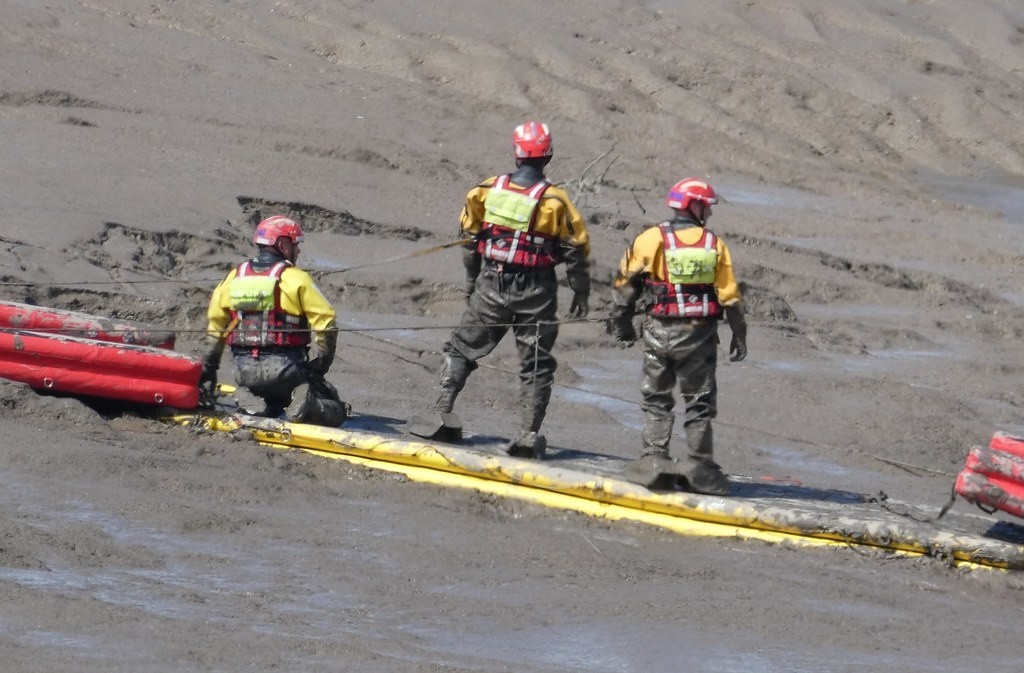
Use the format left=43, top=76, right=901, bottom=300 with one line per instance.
left=568, top=292, right=589, bottom=318
left=197, top=362, right=217, bottom=392
left=728, top=332, right=747, bottom=362
left=464, top=276, right=475, bottom=302
left=607, top=315, right=636, bottom=349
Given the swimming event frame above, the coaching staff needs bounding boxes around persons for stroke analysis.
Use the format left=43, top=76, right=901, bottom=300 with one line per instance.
left=428, top=121, right=590, bottom=439
left=199, top=216, right=347, bottom=429
left=612, top=178, right=748, bottom=464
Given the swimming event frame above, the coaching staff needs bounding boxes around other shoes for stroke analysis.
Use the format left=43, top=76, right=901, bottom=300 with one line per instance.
left=286, top=380, right=316, bottom=422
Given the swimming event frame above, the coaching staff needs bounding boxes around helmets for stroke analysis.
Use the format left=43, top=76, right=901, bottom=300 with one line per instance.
left=665, top=176, right=718, bottom=211
left=511, top=121, right=554, bottom=158
left=253, top=215, right=303, bottom=245
